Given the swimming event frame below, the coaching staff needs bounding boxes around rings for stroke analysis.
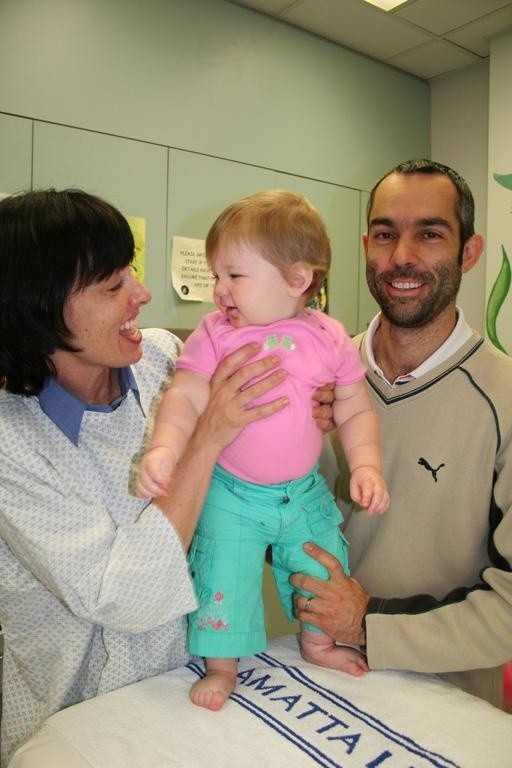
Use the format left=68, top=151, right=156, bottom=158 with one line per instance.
left=305, top=598, right=312, bottom=611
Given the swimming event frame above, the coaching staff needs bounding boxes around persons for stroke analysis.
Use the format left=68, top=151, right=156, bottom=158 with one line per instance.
left=264, top=160, right=512, bottom=712
left=134, top=191, right=390, bottom=710
left=0, top=190, right=337, bottom=768
